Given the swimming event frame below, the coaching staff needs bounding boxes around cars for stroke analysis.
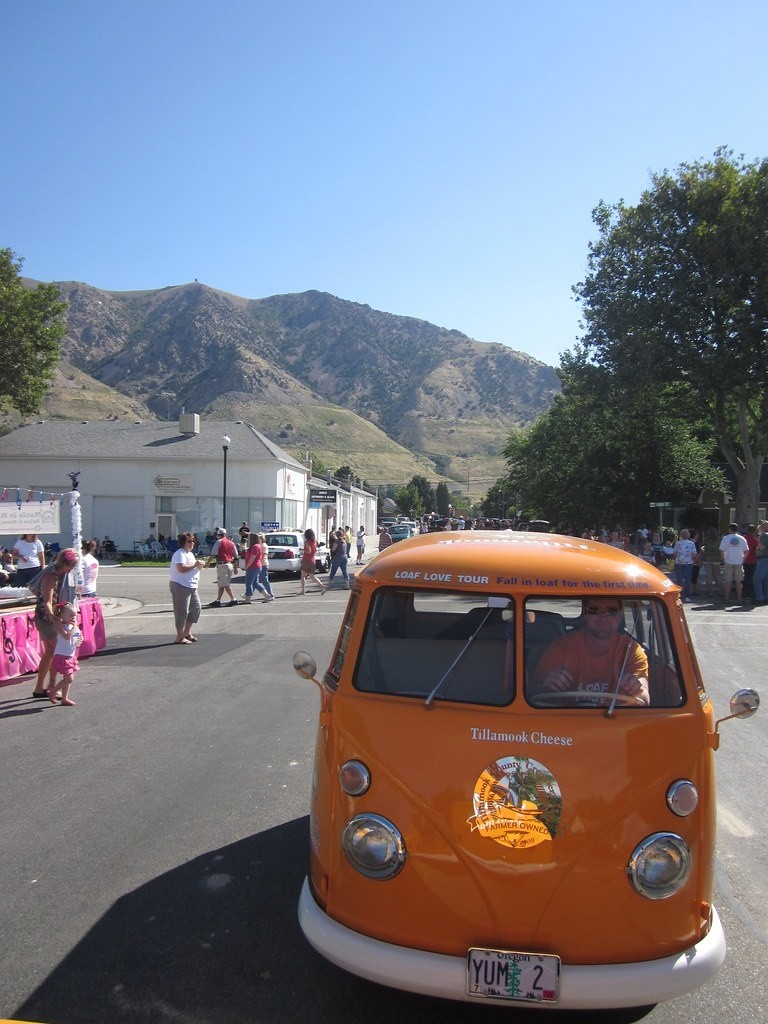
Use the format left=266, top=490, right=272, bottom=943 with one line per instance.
left=377, top=514, right=549, bottom=543
left=291, top=530, right=761, bottom=1011
left=240, top=528, right=332, bottom=576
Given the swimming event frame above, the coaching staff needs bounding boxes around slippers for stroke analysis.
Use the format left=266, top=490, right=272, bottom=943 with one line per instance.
left=689, top=591, right=701, bottom=596
left=175, top=634, right=198, bottom=644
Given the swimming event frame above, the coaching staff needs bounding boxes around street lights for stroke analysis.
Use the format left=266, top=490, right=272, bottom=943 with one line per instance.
left=221, top=433, right=232, bottom=528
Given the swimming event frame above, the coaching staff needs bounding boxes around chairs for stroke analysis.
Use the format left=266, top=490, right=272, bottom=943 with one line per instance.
left=378, top=609, right=579, bottom=705
left=92, top=539, right=169, bottom=561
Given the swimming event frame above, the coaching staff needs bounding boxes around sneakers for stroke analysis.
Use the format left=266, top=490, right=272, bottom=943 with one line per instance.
left=228, top=600, right=238, bottom=605
left=209, top=601, right=221, bottom=607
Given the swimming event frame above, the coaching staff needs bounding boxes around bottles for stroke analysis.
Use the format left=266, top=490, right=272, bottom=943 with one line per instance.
left=72, top=626, right=81, bottom=648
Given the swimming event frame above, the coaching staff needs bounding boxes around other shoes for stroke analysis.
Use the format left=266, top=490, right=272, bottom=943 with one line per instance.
left=46, top=688, right=57, bottom=705
left=240, top=601, right=251, bottom=604
left=268, top=596, right=274, bottom=600
left=296, top=592, right=304, bottom=595
left=241, top=593, right=251, bottom=599
left=321, top=584, right=327, bottom=595
left=262, top=594, right=271, bottom=602
left=724, top=599, right=730, bottom=607
left=61, top=698, right=76, bottom=706
left=752, top=598, right=768, bottom=605
left=33, top=688, right=49, bottom=698
left=685, top=597, right=694, bottom=603
left=745, top=592, right=751, bottom=600
left=737, top=599, right=744, bottom=605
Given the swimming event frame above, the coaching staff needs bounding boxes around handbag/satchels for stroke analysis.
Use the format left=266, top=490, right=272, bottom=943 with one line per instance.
left=755, top=546, right=768, bottom=557
left=666, top=555, right=676, bottom=571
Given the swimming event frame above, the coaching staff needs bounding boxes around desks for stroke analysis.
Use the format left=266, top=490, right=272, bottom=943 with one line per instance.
left=0, top=596, right=107, bottom=682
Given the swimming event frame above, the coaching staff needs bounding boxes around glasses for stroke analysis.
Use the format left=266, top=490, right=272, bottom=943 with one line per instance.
left=187, top=540, right=195, bottom=543
left=582, top=603, right=623, bottom=617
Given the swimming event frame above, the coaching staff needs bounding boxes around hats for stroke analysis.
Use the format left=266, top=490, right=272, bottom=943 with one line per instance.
left=216, top=528, right=226, bottom=534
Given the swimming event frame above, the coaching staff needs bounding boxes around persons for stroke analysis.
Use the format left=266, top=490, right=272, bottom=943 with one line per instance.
left=239, top=521, right=250, bottom=549
left=75, top=540, right=99, bottom=598
left=753, top=521, right=768, bottom=606
left=741, top=524, right=759, bottom=605
left=296, top=529, right=327, bottom=597
left=672, top=529, right=696, bottom=602
left=239, top=532, right=274, bottom=601
left=93, top=538, right=102, bottom=559
left=688, top=530, right=704, bottom=595
left=0, top=549, right=9, bottom=587
left=170, top=532, right=201, bottom=644
left=3, top=552, right=18, bottom=572
left=419, top=515, right=674, bottom=565
left=46, top=602, right=82, bottom=706
left=139, top=540, right=155, bottom=562
left=32, top=548, right=80, bottom=700
left=146, top=534, right=157, bottom=551
left=356, top=526, right=366, bottom=565
left=13, top=534, right=45, bottom=587
left=700, top=527, right=724, bottom=598
left=206, top=528, right=239, bottom=608
left=103, top=536, right=116, bottom=553
left=329, top=526, right=352, bottom=558
left=241, top=533, right=271, bottom=605
left=326, top=531, right=350, bottom=589
left=378, top=527, right=392, bottom=553
left=718, top=523, right=749, bottom=606
left=158, top=526, right=248, bottom=555
left=532, top=600, right=651, bottom=706
